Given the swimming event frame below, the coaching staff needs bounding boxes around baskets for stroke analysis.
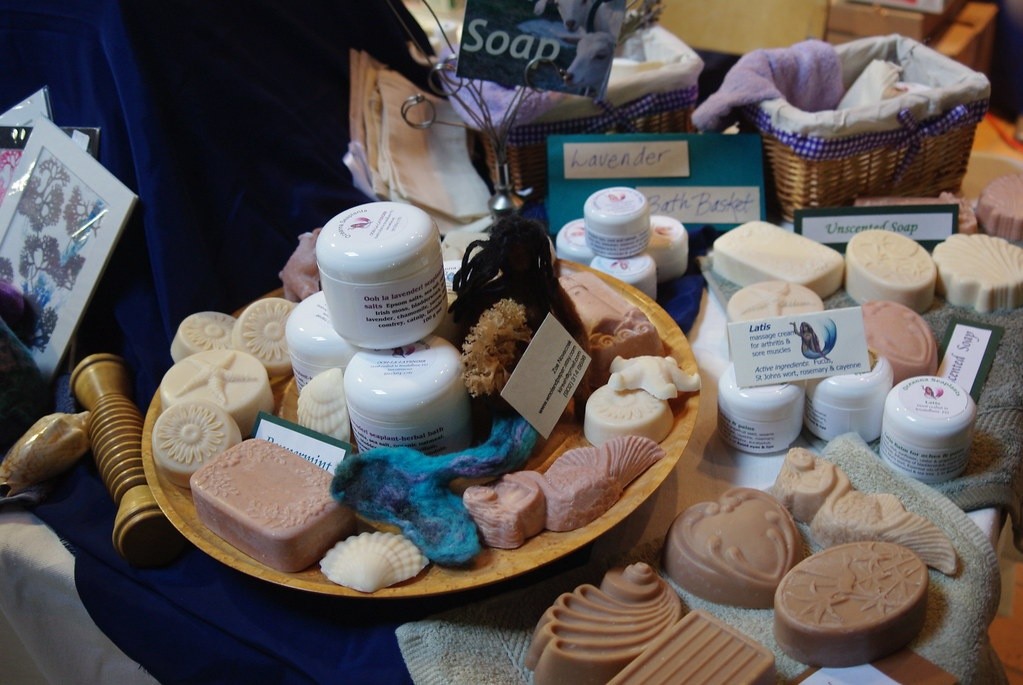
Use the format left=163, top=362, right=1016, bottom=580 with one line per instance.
left=466, top=23, right=704, bottom=200
left=741, top=35, right=991, bottom=219
